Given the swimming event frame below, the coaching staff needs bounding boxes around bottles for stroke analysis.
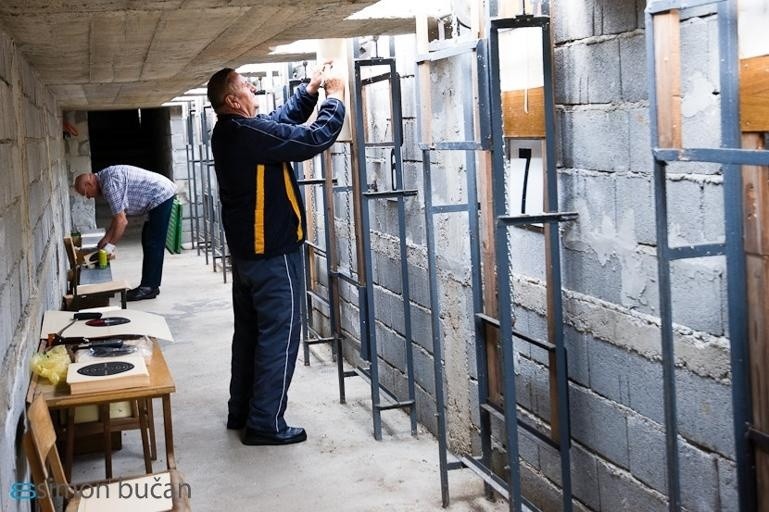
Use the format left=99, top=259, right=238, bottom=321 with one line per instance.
left=98, top=248, right=108, bottom=270
left=70, top=231, right=82, bottom=247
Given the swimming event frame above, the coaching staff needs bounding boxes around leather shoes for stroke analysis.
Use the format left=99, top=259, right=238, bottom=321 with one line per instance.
left=227, top=414, right=306, bottom=446
left=126, top=284, right=160, bottom=301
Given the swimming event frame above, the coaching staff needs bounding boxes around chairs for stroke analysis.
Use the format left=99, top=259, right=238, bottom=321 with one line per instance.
left=20, top=390, right=192, bottom=512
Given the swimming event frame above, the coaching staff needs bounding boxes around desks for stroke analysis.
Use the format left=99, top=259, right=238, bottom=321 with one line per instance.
left=25, top=308, right=178, bottom=511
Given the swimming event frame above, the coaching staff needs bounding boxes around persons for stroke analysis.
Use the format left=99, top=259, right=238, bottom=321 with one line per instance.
left=75, top=164, right=178, bottom=302
left=208, top=59, right=346, bottom=448
